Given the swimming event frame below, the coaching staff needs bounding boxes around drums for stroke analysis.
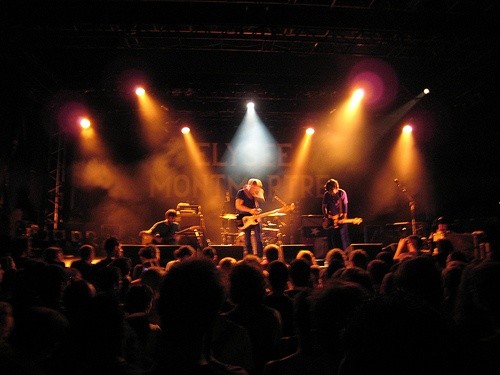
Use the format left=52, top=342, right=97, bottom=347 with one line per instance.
left=222, top=214, right=240, bottom=233
left=235, top=232, right=265, bottom=247
left=266, top=213, right=286, bottom=228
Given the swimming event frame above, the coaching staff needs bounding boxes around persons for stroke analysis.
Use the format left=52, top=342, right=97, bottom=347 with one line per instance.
left=147, top=209, right=180, bottom=245
left=322, top=179, right=348, bottom=251
left=0, top=236, right=500, bottom=374
left=235, top=178, right=266, bottom=259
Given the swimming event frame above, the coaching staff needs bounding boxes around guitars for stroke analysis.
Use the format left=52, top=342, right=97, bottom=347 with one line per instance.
left=236, top=203, right=295, bottom=230
left=139, top=225, right=201, bottom=246
left=321, top=212, right=363, bottom=231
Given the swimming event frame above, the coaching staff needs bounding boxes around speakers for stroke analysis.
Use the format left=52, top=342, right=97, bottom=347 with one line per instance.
left=156, top=245, right=181, bottom=267
left=210, top=245, right=244, bottom=262
left=281, top=244, right=314, bottom=263
left=301, top=215, right=329, bottom=259
left=344, top=243, right=382, bottom=265
left=175, top=214, right=204, bottom=250
left=119, top=244, right=144, bottom=268
